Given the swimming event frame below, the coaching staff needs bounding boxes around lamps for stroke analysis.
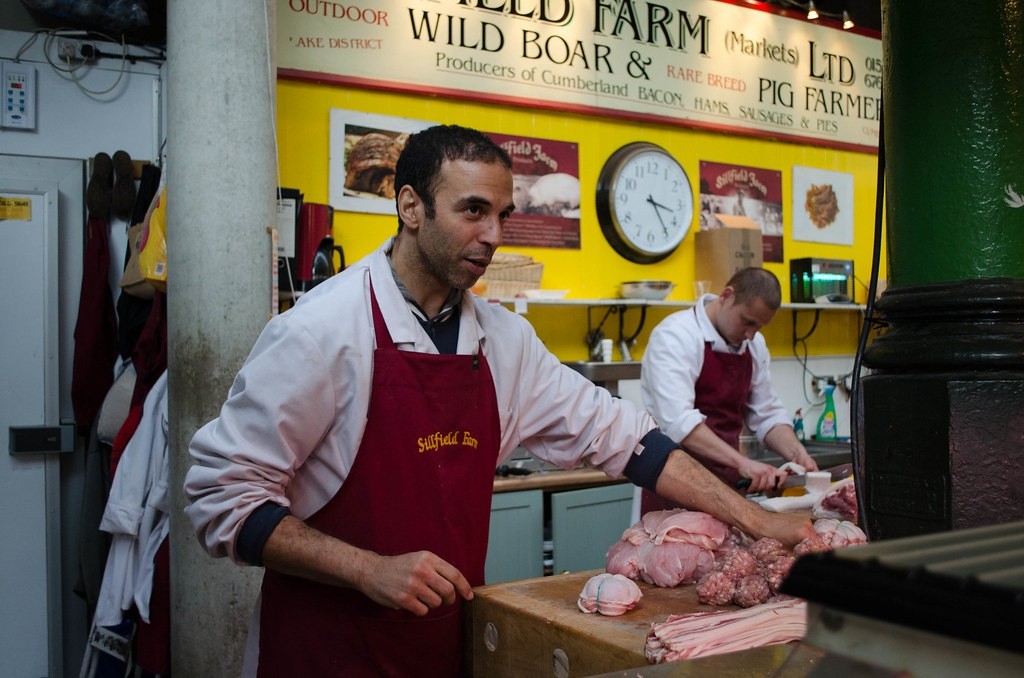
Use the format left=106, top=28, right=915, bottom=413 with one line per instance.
left=842, top=10, right=855, bottom=30
left=802, top=0, right=822, bottom=22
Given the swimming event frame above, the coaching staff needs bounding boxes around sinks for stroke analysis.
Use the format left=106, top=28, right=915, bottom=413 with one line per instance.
left=736, top=435, right=852, bottom=480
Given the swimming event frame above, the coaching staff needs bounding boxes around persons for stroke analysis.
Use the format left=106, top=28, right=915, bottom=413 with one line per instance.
left=627, top=265, right=819, bottom=534
left=179, top=126, right=826, bottom=678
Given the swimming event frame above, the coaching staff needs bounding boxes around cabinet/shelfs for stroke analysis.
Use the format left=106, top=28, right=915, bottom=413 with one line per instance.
left=478, top=481, right=638, bottom=592
left=481, top=293, right=873, bottom=385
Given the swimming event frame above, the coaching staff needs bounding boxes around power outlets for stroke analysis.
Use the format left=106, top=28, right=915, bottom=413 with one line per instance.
left=75, top=39, right=96, bottom=60
left=58, top=37, right=76, bottom=58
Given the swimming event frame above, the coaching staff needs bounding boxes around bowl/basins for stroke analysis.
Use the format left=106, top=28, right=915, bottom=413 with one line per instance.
left=613, top=281, right=673, bottom=299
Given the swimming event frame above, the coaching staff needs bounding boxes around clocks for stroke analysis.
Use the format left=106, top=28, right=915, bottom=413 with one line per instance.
left=595, top=140, right=696, bottom=263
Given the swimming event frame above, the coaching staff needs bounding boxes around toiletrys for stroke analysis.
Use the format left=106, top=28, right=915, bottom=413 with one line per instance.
left=789, top=408, right=804, bottom=442
left=815, top=385, right=838, bottom=441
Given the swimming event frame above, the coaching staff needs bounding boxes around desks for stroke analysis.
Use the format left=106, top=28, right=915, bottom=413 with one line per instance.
left=463, top=560, right=811, bottom=678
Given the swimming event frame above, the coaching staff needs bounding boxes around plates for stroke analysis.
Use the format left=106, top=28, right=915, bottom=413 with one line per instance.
left=523, top=290, right=566, bottom=299
